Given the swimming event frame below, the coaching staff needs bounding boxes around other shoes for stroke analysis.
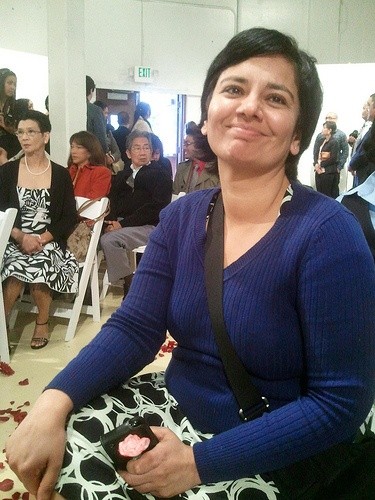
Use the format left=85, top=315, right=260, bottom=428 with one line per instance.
left=111, top=287, right=123, bottom=299
left=71, top=296, right=92, bottom=307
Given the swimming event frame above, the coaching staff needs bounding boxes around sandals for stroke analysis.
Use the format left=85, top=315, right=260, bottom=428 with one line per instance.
left=31, top=321, right=50, bottom=350
left=9, top=346, right=12, bottom=354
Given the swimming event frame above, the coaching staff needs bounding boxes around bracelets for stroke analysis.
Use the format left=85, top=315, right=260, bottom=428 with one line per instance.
left=36, top=235, right=42, bottom=247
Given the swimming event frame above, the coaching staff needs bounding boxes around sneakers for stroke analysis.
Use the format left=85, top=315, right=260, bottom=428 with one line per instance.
left=123, top=276, right=135, bottom=301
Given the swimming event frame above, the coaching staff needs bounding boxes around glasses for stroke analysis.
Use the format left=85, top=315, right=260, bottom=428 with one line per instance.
left=183, top=141, right=195, bottom=147
left=325, top=117, right=336, bottom=119
left=15, top=130, right=41, bottom=137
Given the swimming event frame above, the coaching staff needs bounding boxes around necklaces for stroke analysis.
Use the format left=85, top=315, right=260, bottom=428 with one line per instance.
left=25, top=150, right=50, bottom=175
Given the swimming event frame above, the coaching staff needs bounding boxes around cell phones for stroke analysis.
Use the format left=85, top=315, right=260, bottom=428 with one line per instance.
left=99, top=417, right=159, bottom=470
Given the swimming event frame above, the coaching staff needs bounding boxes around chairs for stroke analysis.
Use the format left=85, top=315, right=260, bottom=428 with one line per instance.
left=101, top=191, right=188, bottom=299
left=0, top=206, right=17, bottom=367
left=16, top=194, right=109, bottom=342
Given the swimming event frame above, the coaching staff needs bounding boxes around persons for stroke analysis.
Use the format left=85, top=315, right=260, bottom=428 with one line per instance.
left=6, top=27, right=375, bottom=500
left=0, top=68, right=222, bottom=350
left=312, top=93, right=375, bottom=200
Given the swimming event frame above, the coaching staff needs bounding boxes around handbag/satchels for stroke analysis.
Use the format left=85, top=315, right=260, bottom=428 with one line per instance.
left=59, top=195, right=111, bottom=262
left=239, top=398, right=375, bottom=500
left=112, top=159, right=124, bottom=173
